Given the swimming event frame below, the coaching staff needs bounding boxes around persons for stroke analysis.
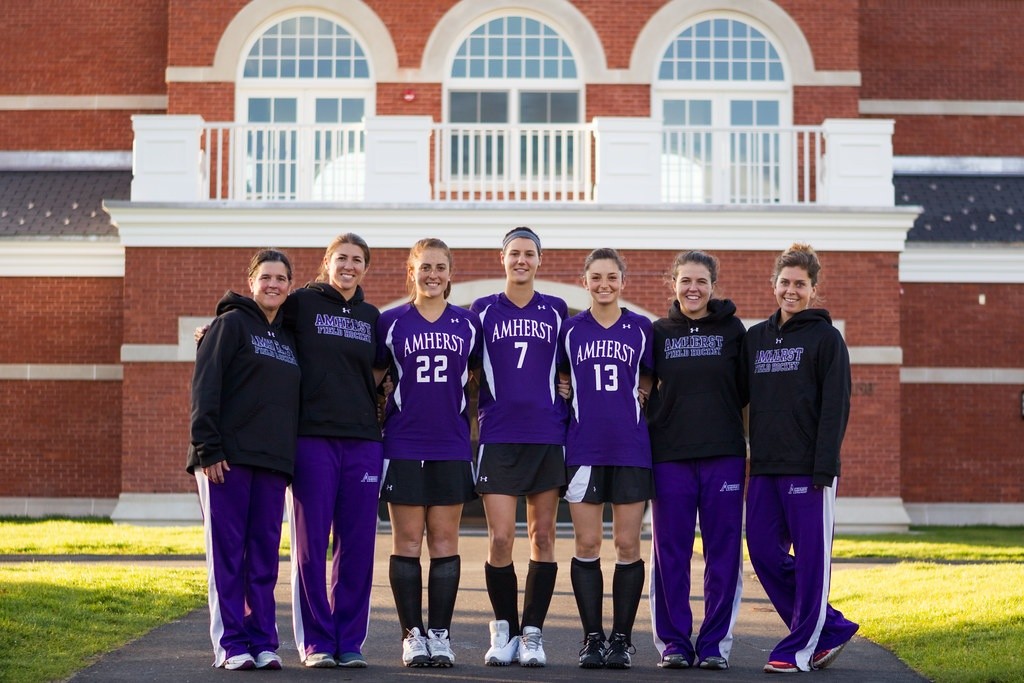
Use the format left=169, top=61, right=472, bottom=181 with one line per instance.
left=556, top=249, right=654, bottom=670
left=281, top=232, right=397, bottom=668
left=745, top=244, right=861, bottom=672
left=375, top=239, right=480, bottom=669
left=634, top=252, right=750, bottom=670
left=469, top=228, right=569, bottom=668
left=187, top=250, right=304, bottom=670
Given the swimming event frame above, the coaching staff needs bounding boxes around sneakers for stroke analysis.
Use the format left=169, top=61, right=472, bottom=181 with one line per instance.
left=402, top=626, right=430, bottom=667
left=578, top=632, right=607, bottom=668
left=517, top=625, right=547, bottom=666
left=661, top=654, right=689, bottom=668
left=810, top=643, right=847, bottom=669
left=700, top=656, right=727, bottom=669
left=305, top=653, right=335, bottom=667
left=484, top=620, right=519, bottom=666
left=604, top=632, right=636, bottom=668
left=425, top=629, right=456, bottom=668
left=336, top=653, right=367, bottom=667
left=223, top=653, right=256, bottom=670
left=256, top=651, right=282, bottom=669
left=764, top=661, right=798, bottom=672
